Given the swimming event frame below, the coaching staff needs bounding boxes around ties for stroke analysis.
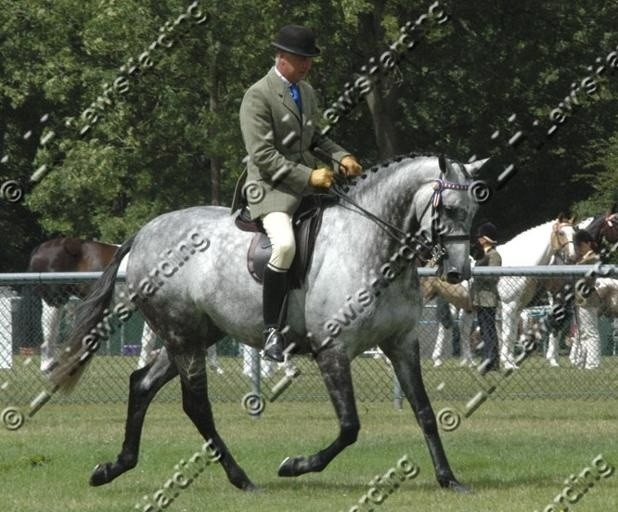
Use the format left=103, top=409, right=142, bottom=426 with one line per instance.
left=290, top=85, right=302, bottom=116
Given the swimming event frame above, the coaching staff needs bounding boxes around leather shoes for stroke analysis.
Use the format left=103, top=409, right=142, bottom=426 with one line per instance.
left=262, top=324, right=292, bottom=363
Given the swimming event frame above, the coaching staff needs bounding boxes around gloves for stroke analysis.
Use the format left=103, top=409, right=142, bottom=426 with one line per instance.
left=309, top=167, right=334, bottom=189
left=339, top=154, right=363, bottom=177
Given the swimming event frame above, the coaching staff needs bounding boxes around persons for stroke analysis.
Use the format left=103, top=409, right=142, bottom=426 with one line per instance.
left=239, top=25, right=365, bottom=363
left=469, top=222, right=502, bottom=371
left=570, top=228, right=604, bottom=369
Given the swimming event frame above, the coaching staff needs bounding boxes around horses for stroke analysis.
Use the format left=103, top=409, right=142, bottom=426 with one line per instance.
left=15, top=229, right=164, bottom=371
left=431, top=212, right=618, bottom=369
left=47, top=149, right=496, bottom=493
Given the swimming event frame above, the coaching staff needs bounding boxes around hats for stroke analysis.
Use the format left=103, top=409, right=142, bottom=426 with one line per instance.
left=475, top=222, right=499, bottom=244
left=268, top=24, right=321, bottom=57
left=568, top=229, right=594, bottom=244
